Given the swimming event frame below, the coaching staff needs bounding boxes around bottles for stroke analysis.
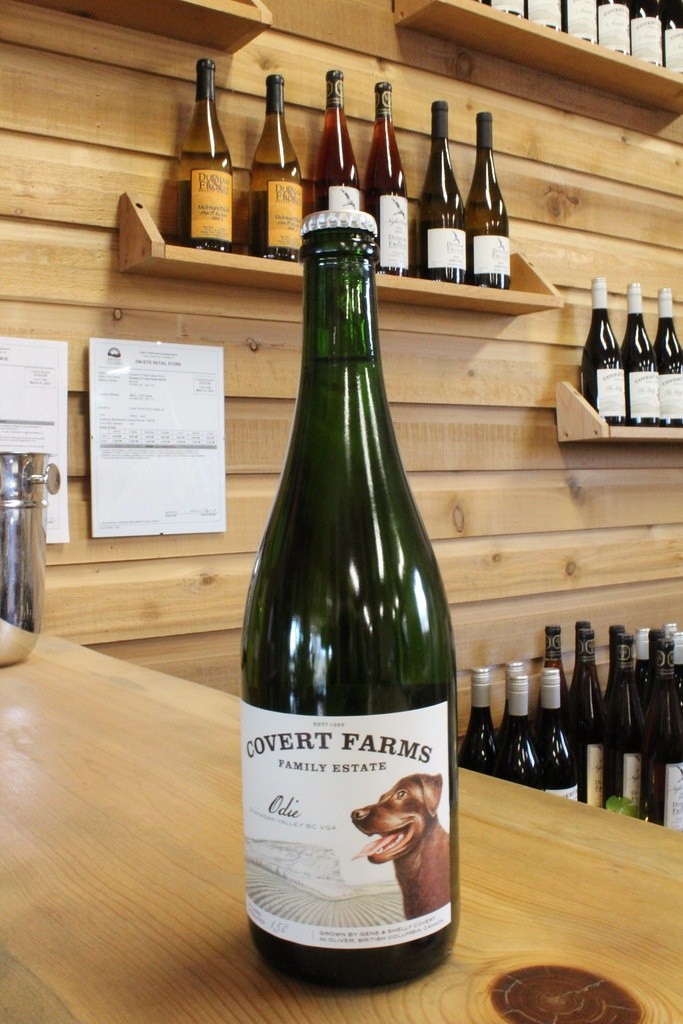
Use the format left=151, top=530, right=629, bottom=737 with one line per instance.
left=535, top=625, right=569, bottom=750
left=465, top=111, right=512, bottom=290
left=314, top=69, right=361, bottom=216
left=175, top=58, right=235, bottom=252
left=570, top=622, right=603, bottom=810
left=362, top=82, right=408, bottom=278
left=238, top=205, right=461, bottom=997
left=634, top=627, right=651, bottom=716
left=414, top=100, right=465, bottom=285
left=489, top=0, right=683, bottom=77
left=621, top=282, right=659, bottom=426
left=504, top=676, right=540, bottom=791
left=604, top=625, right=644, bottom=819
left=538, top=667, right=578, bottom=802
left=458, top=666, right=495, bottom=777
left=580, top=278, right=625, bottom=426
left=249, top=73, right=304, bottom=262
left=497, top=661, right=524, bottom=780
left=652, top=287, right=683, bottom=428
left=661, top=622, right=677, bottom=636
left=643, top=629, right=683, bottom=831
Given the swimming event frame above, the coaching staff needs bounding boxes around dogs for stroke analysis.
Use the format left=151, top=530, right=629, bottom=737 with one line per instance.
left=348, top=773, right=451, bottom=920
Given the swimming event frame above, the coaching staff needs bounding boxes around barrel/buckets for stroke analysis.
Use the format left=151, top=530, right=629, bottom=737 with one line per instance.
left=0, top=451, right=61, bottom=669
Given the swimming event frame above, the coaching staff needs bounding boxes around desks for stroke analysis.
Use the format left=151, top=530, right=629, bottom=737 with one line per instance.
left=0, top=637, right=683, bottom=1024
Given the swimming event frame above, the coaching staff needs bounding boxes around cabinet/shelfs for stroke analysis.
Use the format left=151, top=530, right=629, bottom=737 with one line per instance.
left=32, top=0, right=683, bottom=442
